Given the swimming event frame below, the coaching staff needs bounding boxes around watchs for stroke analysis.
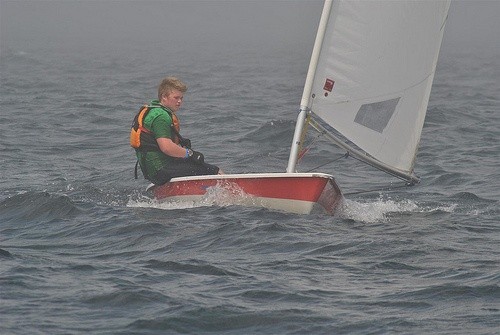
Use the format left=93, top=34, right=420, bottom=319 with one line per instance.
left=186, top=149, right=193, bottom=158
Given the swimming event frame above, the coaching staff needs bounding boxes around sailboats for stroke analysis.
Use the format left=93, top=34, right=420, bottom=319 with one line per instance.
left=145, top=0, right=450, bottom=219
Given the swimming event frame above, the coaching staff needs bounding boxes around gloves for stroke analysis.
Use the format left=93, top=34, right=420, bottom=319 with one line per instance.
left=180, top=138, right=191, bottom=149
left=188, top=151, right=204, bottom=165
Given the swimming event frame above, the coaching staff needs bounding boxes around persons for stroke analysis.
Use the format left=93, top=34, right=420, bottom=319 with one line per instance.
left=132, top=77, right=223, bottom=178
